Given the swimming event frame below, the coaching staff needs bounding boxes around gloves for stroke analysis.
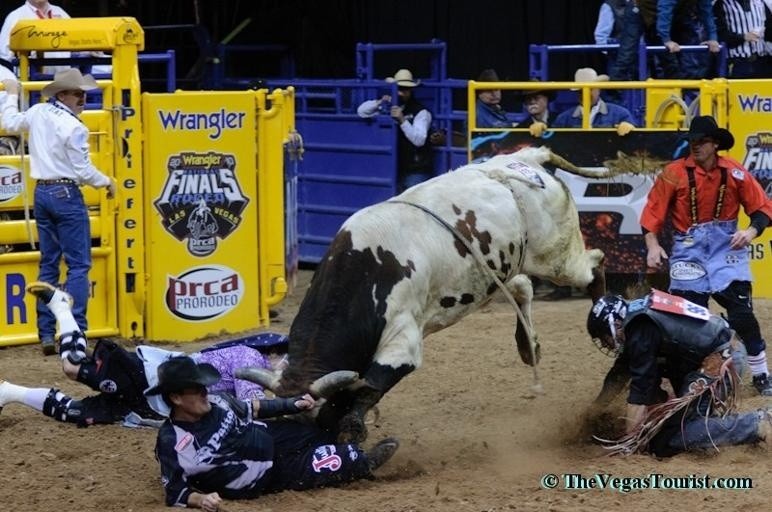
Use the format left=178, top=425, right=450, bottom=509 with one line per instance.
left=273, top=396, right=313, bottom=416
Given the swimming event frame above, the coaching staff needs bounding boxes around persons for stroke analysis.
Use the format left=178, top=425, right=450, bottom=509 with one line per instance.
left=0, top=280, right=291, bottom=430
left=137, top=353, right=403, bottom=512
left=638, top=113, right=771, bottom=396
left=0, top=66, right=117, bottom=359
left=1, top=0, right=105, bottom=76
left=506, top=77, right=564, bottom=141
left=528, top=66, right=644, bottom=140
left=561, top=284, right=768, bottom=459
left=711, top=0, right=770, bottom=80
left=354, top=67, right=436, bottom=201
left=460, top=68, right=515, bottom=147
left=591, top=0, right=658, bottom=80
left=651, top=0, right=723, bottom=80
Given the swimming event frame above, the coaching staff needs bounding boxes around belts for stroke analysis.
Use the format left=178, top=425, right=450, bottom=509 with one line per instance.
left=35, top=178, right=77, bottom=185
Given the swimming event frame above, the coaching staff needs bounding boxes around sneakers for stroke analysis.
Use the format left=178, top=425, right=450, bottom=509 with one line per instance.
left=363, top=435, right=402, bottom=473
left=23, top=280, right=76, bottom=305
left=41, top=337, right=97, bottom=358
left=755, top=406, right=772, bottom=447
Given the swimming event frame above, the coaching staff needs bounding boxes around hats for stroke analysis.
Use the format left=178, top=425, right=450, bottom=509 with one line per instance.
left=677, top=115, right=734, bottom=152
left=39, top=68, right=99, bottom=98
left=752, top=370, right=772, bottom=396
left=563, top=66, right=610, bottom=95
left=512, top=75, right=561, bottom=104
left=143, top=353, right=221, bottom=398
left=472, top=66, right=506, bottom=91
left=382, top=68, right=424, bottom=90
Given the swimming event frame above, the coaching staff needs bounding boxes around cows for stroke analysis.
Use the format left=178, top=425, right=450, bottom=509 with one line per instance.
left=231, top=146, right=663, bottom=448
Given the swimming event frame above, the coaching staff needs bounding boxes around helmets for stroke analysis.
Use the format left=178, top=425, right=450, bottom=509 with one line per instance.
left=584, top=292, right=630, bottom=340
